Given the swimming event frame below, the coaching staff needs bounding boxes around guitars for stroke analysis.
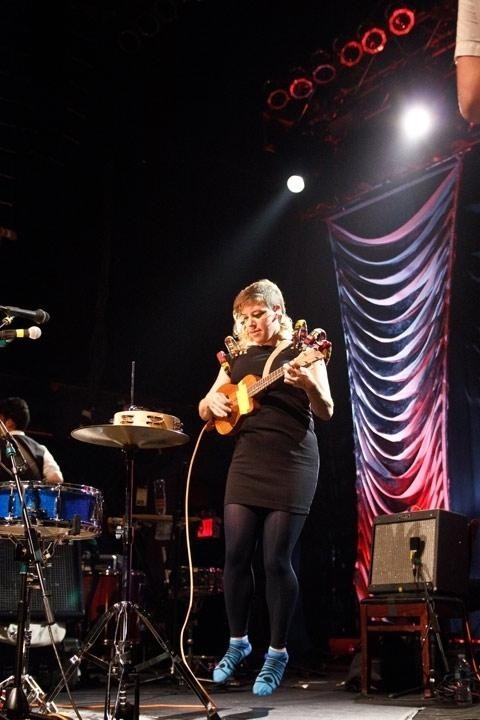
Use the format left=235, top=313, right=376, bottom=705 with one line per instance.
left=212, top=345, right=324, bottom=436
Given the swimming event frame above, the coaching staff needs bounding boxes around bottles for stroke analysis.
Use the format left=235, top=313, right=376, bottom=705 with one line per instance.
left=454, top=655, right=472, bottom=706
left=154, top=479, right=166, bottom=517
left=135, top=469, right=150, bottom=513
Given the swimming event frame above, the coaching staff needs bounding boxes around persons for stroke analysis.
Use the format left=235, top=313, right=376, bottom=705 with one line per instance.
left=0, top=399, right=63, bottom=484
left=455, top=0, right=480, bottom=126
left=198, top=280, right=335, bottom=696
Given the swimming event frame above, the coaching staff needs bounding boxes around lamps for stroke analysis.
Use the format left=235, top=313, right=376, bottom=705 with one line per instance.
left=267, top=7, right=414, bottom=109
left=286, top=166, right=315, bottom=194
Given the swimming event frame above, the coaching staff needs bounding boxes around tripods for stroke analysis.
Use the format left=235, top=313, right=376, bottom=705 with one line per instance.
left=123, top=541, right=241, bottom=690
left=0, top=533, right=62, bottom=717
left=36, top=449, right=223, bottom=720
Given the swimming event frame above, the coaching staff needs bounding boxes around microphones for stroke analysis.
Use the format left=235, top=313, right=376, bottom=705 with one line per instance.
left=409, top=537, right=421, bottom=577
left=0, top=305, right=50, bottom=324
left=0, top=325, right=42, bottom=340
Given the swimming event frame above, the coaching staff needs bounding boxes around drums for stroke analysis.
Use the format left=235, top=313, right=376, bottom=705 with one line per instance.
left=76, top=554, right=133, bottom=576
left=82, top=572, right=143, bottom=646
left=1, top=480, right=105, bottom=543
left=170, top=567, right=225, bottom=595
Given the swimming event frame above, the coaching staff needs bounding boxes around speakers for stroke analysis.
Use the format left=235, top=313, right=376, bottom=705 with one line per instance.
left=1, top=538, right=85, bottom=617
left=368, top=508, right=473, bottom=595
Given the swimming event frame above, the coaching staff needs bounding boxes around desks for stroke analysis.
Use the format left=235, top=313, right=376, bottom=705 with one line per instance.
left=358, top=594, right=465, bottom=701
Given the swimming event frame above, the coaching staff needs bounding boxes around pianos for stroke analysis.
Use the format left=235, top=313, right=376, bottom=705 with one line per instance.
left=107, top=513, right=221, bottom=535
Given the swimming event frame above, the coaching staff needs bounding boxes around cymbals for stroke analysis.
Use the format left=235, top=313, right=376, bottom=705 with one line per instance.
left=71, top=425, right=188, bottom=450
